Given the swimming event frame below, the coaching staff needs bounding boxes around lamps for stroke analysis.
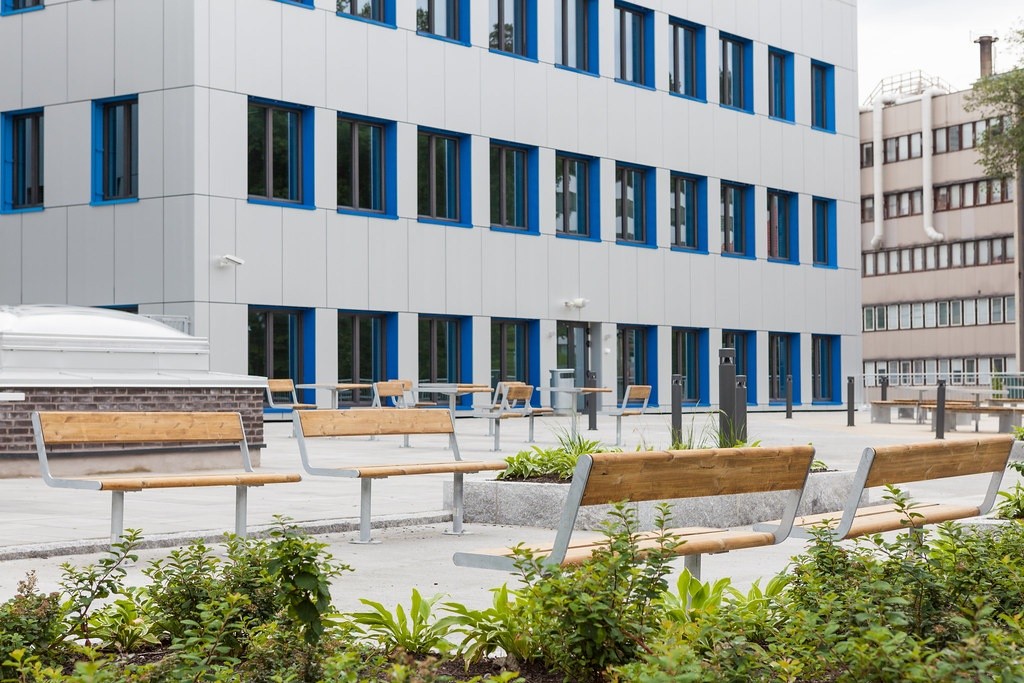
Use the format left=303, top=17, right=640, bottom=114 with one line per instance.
left=218, top=252, right=243, bottom=271
left=565, top=297, right=590, bottom=311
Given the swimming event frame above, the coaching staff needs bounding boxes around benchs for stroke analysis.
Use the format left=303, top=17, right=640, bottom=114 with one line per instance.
left=389, top=379, right=438, bottom=408
left=373, top=381, right=406, bottom=408
left=452, top=444, right=816, bottom=583
left=265, top=378, right=317, bottom=410
left=473, top=386, right=533, bottom=450
left=471, top=381, right=502, bottom=409
left=597, top=385, right=651, bottom=446
left=293, top=407, right=509, bottom=545
left=502, top=381, right=554, bottom=416
left=32, top=410, right=301, bottom=566
left=870, top=398, right=1024, bottom=433
left=750, top=431, right=1015, bottom=544
left=536, top=385, right=612, bottom=442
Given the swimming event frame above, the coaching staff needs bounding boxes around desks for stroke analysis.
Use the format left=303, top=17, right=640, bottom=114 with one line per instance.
left=419, top=383, right=489, bottom=387
left=296, top=383, right=374, bottom=409
left=416, top=387, right=494, bottom=450
